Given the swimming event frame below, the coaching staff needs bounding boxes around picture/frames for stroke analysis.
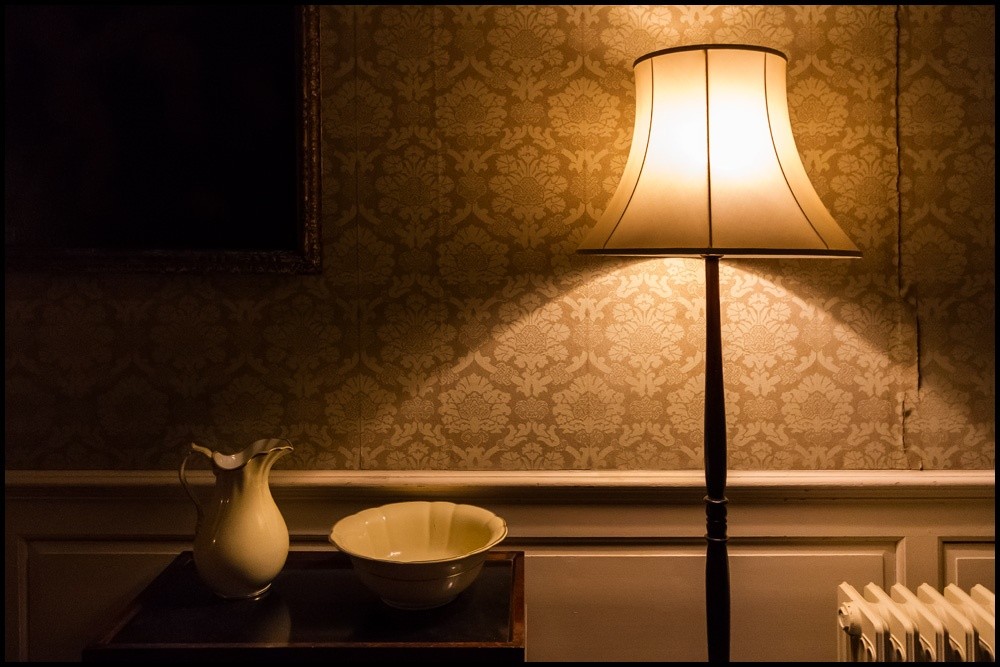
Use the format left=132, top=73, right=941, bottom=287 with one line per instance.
left=3, top=1, right=324, bottom=276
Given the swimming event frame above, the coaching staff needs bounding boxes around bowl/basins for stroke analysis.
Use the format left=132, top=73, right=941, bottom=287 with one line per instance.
left=329, top=500, right=507, bottom=610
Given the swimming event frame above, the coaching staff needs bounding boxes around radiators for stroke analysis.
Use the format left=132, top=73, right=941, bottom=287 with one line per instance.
left=835, top=582, right=996, bottom=662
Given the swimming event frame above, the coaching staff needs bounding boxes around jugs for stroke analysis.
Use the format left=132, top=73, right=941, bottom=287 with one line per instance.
left=177, top=427, right=297, bottom=601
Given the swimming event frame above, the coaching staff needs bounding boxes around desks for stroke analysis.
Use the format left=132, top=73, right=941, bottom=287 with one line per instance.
left=84, top=551, right=527, bottom=664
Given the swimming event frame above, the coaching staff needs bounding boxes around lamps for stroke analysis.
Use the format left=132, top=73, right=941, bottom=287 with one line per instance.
left=576, top=44, right=864, bottom=662
